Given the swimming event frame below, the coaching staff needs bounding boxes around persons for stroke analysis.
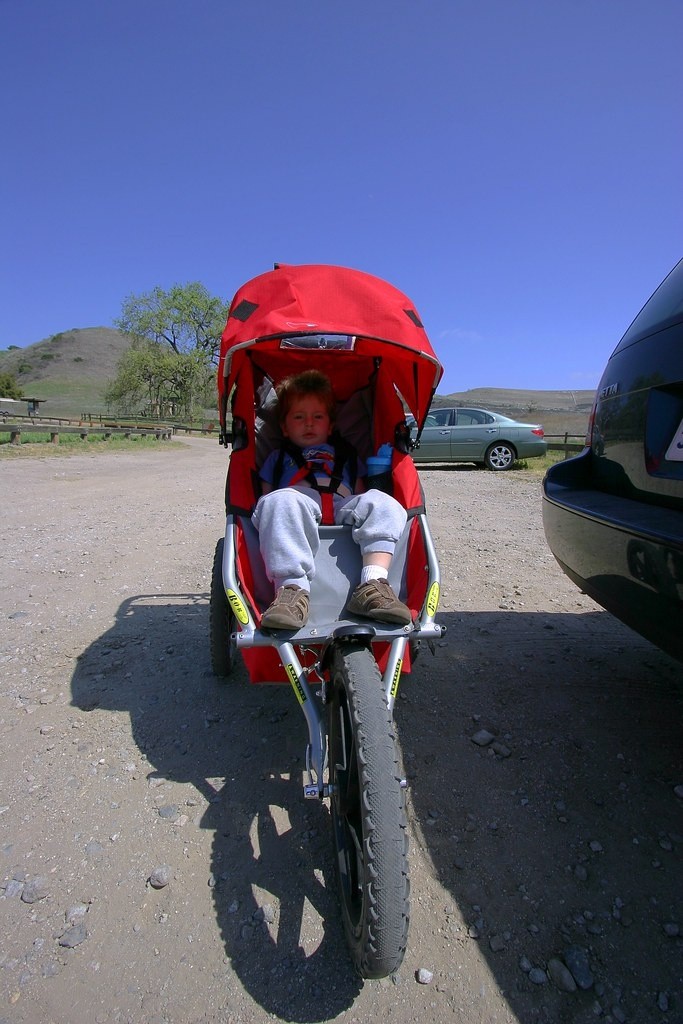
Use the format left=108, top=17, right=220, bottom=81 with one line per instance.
left=251, top=370, right=412, bottom=631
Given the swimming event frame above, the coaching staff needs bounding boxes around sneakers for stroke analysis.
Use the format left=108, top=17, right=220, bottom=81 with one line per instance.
left=261, top=584, right=309, bottom=629
left=346, top=578, right=412, bottom=623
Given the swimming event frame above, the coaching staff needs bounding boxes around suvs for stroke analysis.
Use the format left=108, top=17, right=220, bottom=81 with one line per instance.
left=539, top=256, right=682, bottom=657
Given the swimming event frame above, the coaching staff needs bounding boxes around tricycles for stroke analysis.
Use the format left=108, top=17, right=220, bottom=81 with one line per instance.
left=209, top=262, right=449, bottom=981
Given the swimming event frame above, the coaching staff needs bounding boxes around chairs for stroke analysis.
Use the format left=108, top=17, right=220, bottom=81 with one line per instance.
left=436, top=415, right=443, bottom=426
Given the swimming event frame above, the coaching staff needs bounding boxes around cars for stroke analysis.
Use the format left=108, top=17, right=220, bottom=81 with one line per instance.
left=404, top=406, right=548, bottom=471
